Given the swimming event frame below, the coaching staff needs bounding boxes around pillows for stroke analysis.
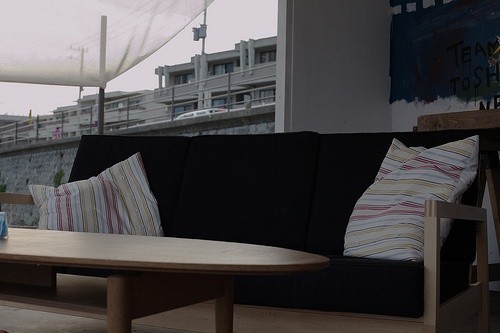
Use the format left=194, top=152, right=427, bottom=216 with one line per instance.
left=28, top=151, right=164, bottom=237
left=342, top=134, right=480, bottom=262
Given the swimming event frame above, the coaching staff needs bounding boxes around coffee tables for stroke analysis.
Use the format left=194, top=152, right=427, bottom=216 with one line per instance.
left=0, top=225, right=331, bottom=333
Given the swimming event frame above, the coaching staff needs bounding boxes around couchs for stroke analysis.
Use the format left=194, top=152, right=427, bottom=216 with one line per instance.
left=0, top=130, right=492, bottom=333
